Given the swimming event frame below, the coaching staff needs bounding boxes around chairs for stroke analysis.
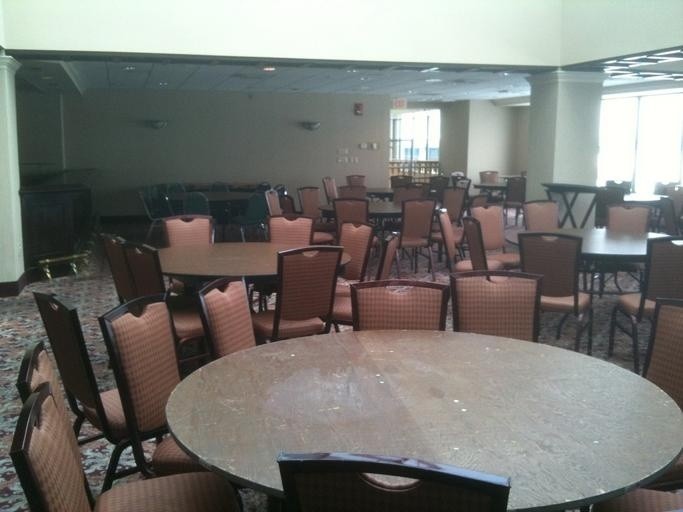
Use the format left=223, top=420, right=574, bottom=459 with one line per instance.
left=296, top=186, right=324, bottom=224
left=429, top=175, right=451, bottom=193
left=431, top=188, right=467, bottom=262
left=346, top=174, right=366, bottom=186
left=431, top=194, right=489, bottom=269
left=503, top=229, right=648, bottom=258
left=605, top=203, right=651, bottom=236
left=210, top=181, right=230, bottom=193
left=350, top=279, right=451, bottom=331
left=593, top=296, right=682, bottom=492
left=461, top=216, right=489, bottom=272
left=592, top=486, right=682, bottom=512
left=255, top=181, right=269, bottom=193
left=451, top=174, right=473, bottom=194
left=391, top=174, right=415, bottom=188
left=273, top=183, right=289, bottom=201
left=101, top=230, right=136, bottom=304
left=331, top=233, right=401, bottom=333
left=501, top=175, right=528, bottom=230
left=250, top=243, right=345, bottom=346
left=437, top=207, right=503, bottom=275
left=333, top=219, right=376, bottom=298
left=193, top=276, right=257, bottom=361
left=8, top=381, right=245, bottom=512
left=179, top=190, right=213, bottom=215
left=323, top=176, right=338, bottom=207
left=520, top=199, right=560, bottom=232
left=264, top=188, right=282, bottom=219
left=15, top=340, right=86, bottom=483
left=277, top=452, right=513, bottom=512
left=467, top=202, right=522, bottom=269
left=32, top=290, right=163, bottom=495
left=336, top=185, right=370, bottom=198
left=279, top=195, right=303, bottom=218
left=165, top=180, right=188, bottom=199
left=392, top=182, right=424, bottom=205
left=134, top=185, right=164, bottom=245
left=395, top=198, right=438, bottom=282
left=227, top=191, right=270, bottom=243
left=450, top=270, right=546, bottom=343
left=97, top=293, right=208, bottom=480
left=125, top=242, right=207, bottom=360
left=332, top=197, right=383, bottom=259
left=518, top=231, right=595, bottom=357
left=608, top=236, right=683, bottom=375
left=161, top=213, right=216, bottom=247
left=171, top=295, right=177, bottom=305
left=266, top=214, right=318, bottom=246
left=156, top=191, right=177, bottom=220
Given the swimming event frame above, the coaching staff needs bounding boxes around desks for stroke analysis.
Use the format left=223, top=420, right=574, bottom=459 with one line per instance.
left=365, top=186, right=397, bottom=198
left=318, top=200, right=403, bottom=215
left=155, top=241, right=350, bottom=278
left=474, top=183, right=507, bottom=203
left=164, top=329, right=683, bottom=512
left=541, top=184, right=602, bottom=233
